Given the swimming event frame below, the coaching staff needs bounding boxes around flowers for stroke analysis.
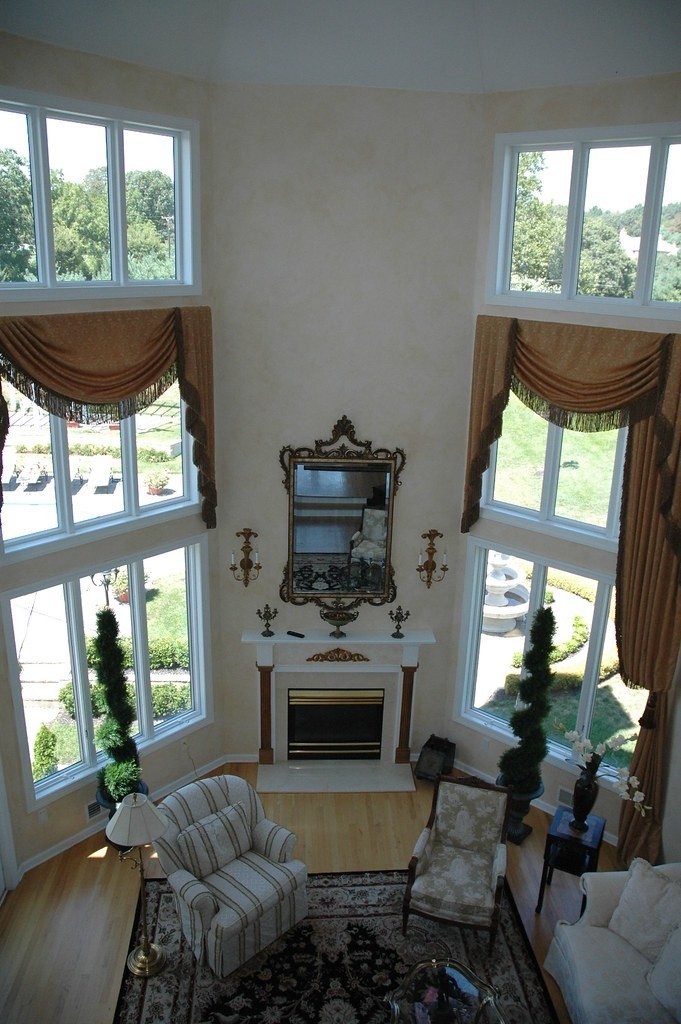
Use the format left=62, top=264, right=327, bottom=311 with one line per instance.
left=552, top=718, right=653, bottom=818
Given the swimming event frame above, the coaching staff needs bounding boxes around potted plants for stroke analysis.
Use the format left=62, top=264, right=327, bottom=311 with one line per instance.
left=93, top=610, right=149, bottom=855
left=495, top=606, right=555, bottom=845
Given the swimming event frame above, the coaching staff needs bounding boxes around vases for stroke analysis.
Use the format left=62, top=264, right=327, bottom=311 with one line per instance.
left=568, top=775, right=599, bottom=832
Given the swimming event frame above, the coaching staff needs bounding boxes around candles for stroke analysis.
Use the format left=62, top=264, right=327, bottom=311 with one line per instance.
left=255, top=551, right=260, bottom=563
left=419, top=553, right=423, bottom=565
left=231, top=553, right=235, bottom=564
left=442, top=553, right=447, bottom=566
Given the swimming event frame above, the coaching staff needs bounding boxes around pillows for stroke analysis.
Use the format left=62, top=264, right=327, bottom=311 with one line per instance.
left=644, top=927, right=681, bottom=1023
left=608, top=859, right=681, bottom=963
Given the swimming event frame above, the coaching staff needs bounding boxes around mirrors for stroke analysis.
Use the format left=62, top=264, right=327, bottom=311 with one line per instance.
left=279, top=415, right=406, bottom=611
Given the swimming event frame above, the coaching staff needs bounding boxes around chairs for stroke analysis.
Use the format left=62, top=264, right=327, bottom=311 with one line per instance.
left=402, top=776, right=513, bottom=957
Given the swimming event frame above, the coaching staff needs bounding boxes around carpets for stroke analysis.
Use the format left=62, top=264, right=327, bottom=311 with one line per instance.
left=111, top=870, right=560, bottom=1024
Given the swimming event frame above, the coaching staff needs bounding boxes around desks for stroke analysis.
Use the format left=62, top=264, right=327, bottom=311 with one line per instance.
left=534, top=806, right=606, bottom=919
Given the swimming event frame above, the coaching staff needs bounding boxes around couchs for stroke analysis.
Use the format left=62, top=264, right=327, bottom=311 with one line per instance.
left=152, top=774, right=309, bottom=981
left=543, top=857, right=681, bottom=1024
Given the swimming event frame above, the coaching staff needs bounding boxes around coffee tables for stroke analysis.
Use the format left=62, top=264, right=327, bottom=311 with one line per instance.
left=382, top=959, right=507, bottom=1024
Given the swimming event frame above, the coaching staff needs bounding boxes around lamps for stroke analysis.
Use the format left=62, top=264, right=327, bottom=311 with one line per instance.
left=104, top=791, right=169, bottom=978
left=256, top=604, right=278, bottom=638
left=388, top=606, right=410, bottom=639
